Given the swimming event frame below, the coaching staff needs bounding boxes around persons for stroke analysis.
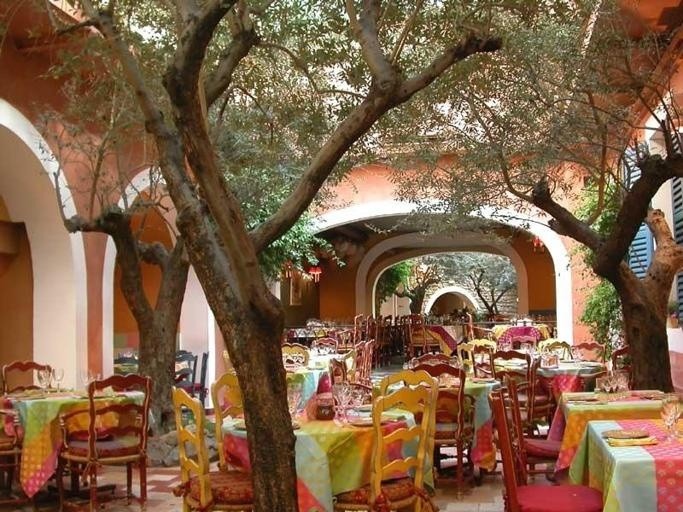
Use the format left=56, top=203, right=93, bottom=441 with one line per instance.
left=461, top=306, right=474, bottom=340
left=453, top=309, right=462, bottom=316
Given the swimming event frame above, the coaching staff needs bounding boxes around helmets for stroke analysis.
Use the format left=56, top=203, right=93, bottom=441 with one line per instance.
left=350, top=419, right=386, bottom=427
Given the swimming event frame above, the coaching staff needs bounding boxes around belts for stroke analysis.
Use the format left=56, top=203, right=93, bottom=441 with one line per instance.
left=570, top=347, right=584, bottom=370
left=659, top=395, right=683, bottom=447
left=36, top=368, right=93, bottom=396
left=601, top=372, right=628, bottom=400
left=283, top=353, right=304, bottom=369
left=520, top=343, right=531, bottom=353
left=334, top=381, right=362, bottom=423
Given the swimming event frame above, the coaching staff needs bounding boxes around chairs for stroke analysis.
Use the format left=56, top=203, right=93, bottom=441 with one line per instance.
left=0, top=310, right=608, bottom=512
